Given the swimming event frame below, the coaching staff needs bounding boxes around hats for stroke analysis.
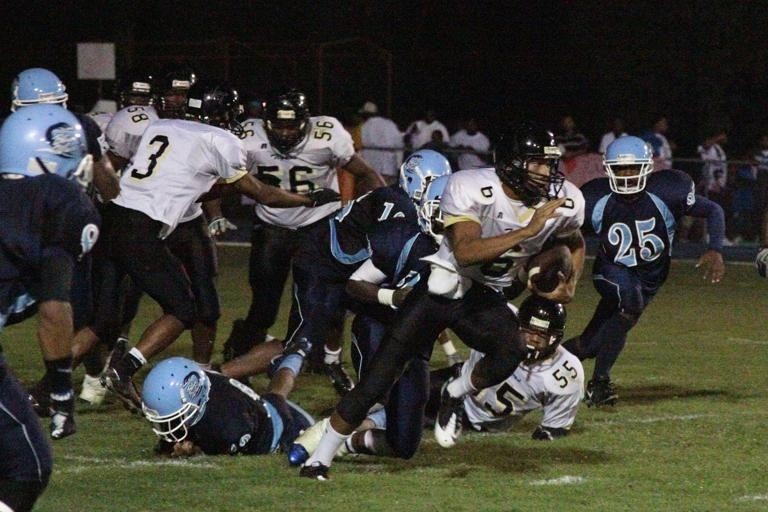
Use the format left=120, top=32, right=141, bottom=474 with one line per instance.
left=357, top=101, right=378, bottom=112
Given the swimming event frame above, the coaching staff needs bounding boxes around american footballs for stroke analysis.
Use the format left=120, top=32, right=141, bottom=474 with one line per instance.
left=527, top=244, right=573, bottom=293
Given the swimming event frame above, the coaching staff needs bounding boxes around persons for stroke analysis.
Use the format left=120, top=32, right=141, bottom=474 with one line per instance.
left=1, top=69, right=585, bottom=510
left=561, top=136, right=725, bottom=406
left=597, top=118, right=768, bottom=248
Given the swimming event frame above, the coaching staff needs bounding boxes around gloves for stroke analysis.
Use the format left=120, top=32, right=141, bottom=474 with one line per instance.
left=206, top=217, right=237, bottom=236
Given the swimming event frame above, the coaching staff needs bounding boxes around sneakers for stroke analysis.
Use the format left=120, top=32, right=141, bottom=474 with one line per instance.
left=585, top=377, right=620, bottom=410
left=28, top=338, right=145, bottom=440
left=434, top=379, right=465, bottom=449
left=224, top=319, right=355, bottom=395
left=289, top=417, right=350, bottom=480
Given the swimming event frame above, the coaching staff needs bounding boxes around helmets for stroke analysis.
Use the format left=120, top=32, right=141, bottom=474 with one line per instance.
left=109, top=63, right=311, bottom=159
left=516, top=293, right=567, bottom=364
left=492, top=121, right=566, bottom=205
left=596, top=132, right=672, bottom=195
left=400, top=148, right=452, bottom=208
left=142, top=354, right=212, bottom=446
left=0, top=104, right=93, bottom=193
left=10, top=64, right=69, bottom=115
